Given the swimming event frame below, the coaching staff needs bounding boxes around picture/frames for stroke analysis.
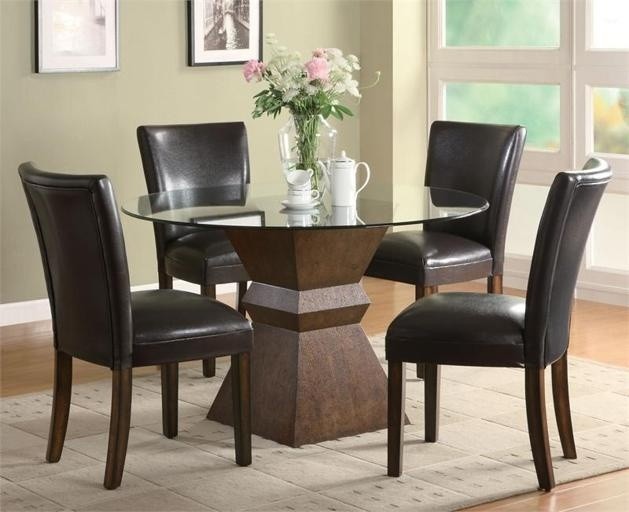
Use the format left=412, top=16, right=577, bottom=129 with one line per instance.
left=188, top=0, right=262, bottom=67
left=34, top=0, right=120, bottom=74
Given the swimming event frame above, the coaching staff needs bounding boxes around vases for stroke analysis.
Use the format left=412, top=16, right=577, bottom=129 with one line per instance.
left=280, top=114, right=338, bottom=204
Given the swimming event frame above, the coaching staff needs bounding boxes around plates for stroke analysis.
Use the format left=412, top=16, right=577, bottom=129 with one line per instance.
left=280, top=199, right=321, bottom=210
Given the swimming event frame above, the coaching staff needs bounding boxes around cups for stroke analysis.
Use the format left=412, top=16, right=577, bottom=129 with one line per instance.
left=285, top=168, right=313, bottom=188
left=286, top=188, right=320, bottom=204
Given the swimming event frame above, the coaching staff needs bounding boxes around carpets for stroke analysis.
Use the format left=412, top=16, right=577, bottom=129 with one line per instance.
left=2, top=332, right=629, bottom=512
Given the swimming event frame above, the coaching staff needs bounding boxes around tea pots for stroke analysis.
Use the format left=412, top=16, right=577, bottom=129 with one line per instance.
left=316, top=150, right=371, bottom=207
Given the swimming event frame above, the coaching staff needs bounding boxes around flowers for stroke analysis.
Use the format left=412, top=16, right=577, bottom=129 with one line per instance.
left=244, top=34, right=381, bottom=190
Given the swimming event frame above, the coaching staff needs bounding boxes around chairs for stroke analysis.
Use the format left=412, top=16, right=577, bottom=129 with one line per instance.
left=365, top=121, right=526, bottom=379
left=18, top=162, right=252, bottom=489
left=137, top=121, right=251, bottom=378
left=384, top=158, right=611, bottom=491
